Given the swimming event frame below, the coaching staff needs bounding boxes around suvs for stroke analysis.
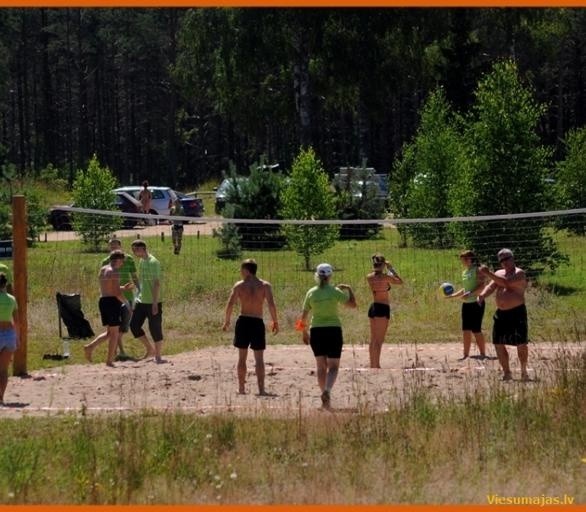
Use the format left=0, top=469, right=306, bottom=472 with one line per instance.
left=213, top=178, right=251, bottom=214
left=47, top=186, right=183, bottom=231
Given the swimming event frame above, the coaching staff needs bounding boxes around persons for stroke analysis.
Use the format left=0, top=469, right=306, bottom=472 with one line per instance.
left=0, top=262, right=14, bottom=297
left=98, top=237, right=137, bottom=360
left=83, top=248, right=136, bottom=368
left=0, top=272, right=19, bottom=405
left=137, top=180, right=154, bottom=226
left=477, top=248, right=530, bottom=384
left=298, top=261, right=357, bottom=408
left=443, top=250, right=488, bottom=361
left=128, top=240, right=166, bottom=364
left=366, top=252, right=404, bottom=369
left=222, top=257, right=280, bottom=396
left=168, top=199, right=186, bottom=256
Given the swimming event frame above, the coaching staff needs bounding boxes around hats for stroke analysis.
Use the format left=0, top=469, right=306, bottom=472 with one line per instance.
left=372, top=254, right=386, bottom=265
left=317, top=263, right=334, bottom=277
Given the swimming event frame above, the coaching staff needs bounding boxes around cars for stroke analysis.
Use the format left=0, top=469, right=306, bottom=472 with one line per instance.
left=176, top=191, right=203, bottom=217
left=338, top=181, right=389, bottom=212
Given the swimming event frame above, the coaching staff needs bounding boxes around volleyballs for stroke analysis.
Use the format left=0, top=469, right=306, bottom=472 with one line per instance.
left=440, top=282, right=452, bottom=295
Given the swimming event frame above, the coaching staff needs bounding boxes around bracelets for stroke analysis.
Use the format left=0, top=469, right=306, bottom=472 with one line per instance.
left=225, top=320, right=232, bottom=327
left=273, top=322, right=278, bottom=325
left=296, top=319, right=308, bottom=331
left=388, top=267, right=396, bottom=276
left=123, top=300, right=131, bottom=310
left=465, top=290, right=473, bottom=297
